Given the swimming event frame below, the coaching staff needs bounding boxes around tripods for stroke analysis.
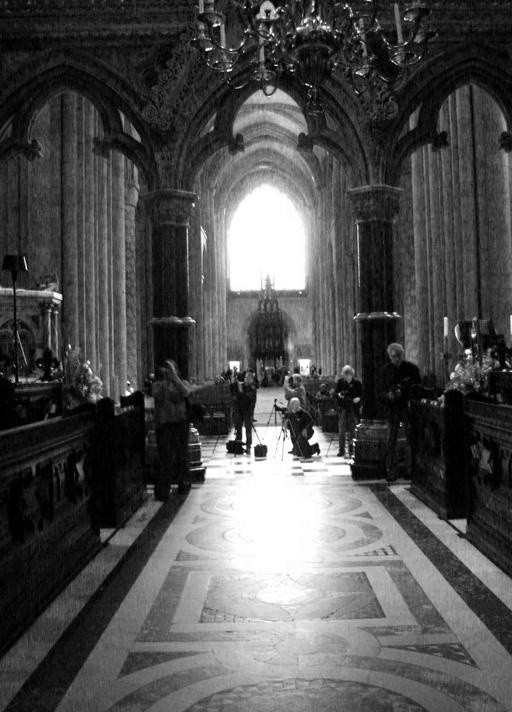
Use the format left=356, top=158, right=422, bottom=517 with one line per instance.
left=273, top=418, right=303, bottom=461
left=266, top=400, right=285, bottom=426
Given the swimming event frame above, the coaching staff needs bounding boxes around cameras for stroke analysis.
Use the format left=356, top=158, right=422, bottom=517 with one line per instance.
left=157, top=366, right=167, bottom=377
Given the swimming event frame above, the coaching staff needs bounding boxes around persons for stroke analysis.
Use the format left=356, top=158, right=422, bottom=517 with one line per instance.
left=149, top=359, right=193, bottom=498
left=215, top=362, right=362, bottom=458
left=372, top=345, right=421, bottom=482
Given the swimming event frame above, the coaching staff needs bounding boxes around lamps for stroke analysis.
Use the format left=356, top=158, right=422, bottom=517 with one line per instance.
left=186, top=0, right=445, bottom=122
